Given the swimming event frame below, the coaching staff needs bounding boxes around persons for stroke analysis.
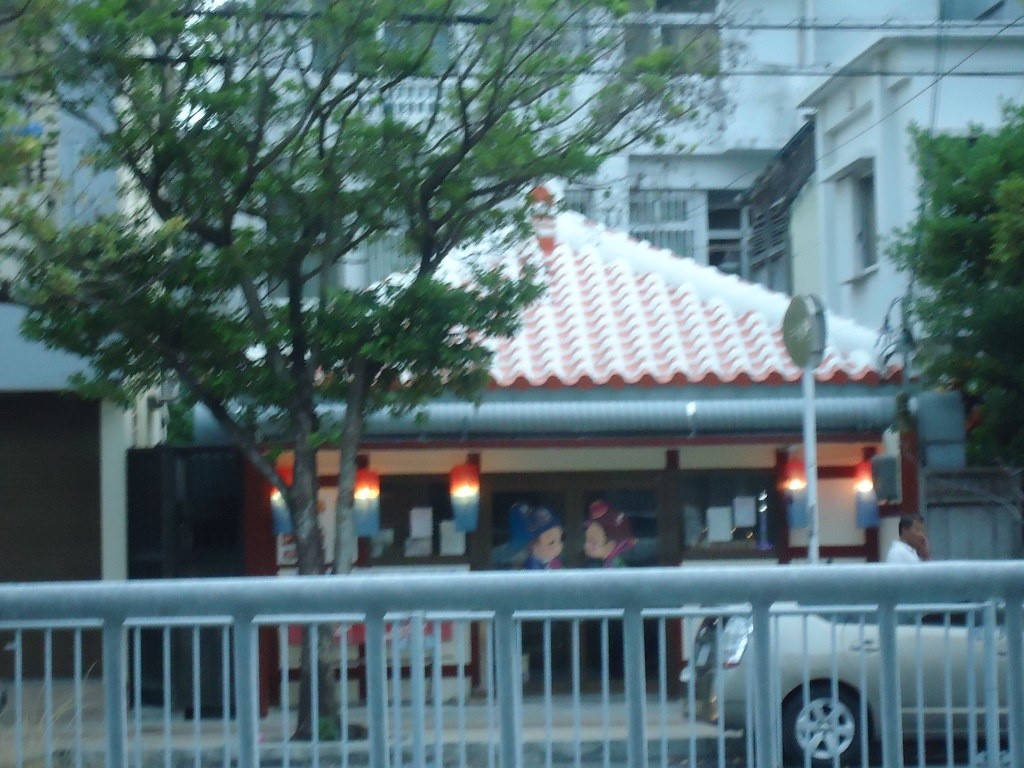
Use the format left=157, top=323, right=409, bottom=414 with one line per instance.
left=884, top=512, right=931, bottom=563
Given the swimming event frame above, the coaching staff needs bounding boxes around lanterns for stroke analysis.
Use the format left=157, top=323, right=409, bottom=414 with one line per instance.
left=781, top=459, right=809, bottom=529
left=449, top=461, right=480, bottom=533
left=351, top=469, right=382, bottom=538
left=855, top=458, right=884, bottom=528
left=268, top=465, right=297, bottom=534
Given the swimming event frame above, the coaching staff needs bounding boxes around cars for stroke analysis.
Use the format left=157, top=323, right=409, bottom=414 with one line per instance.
left=681, top=602, right=1024, bottom=768
